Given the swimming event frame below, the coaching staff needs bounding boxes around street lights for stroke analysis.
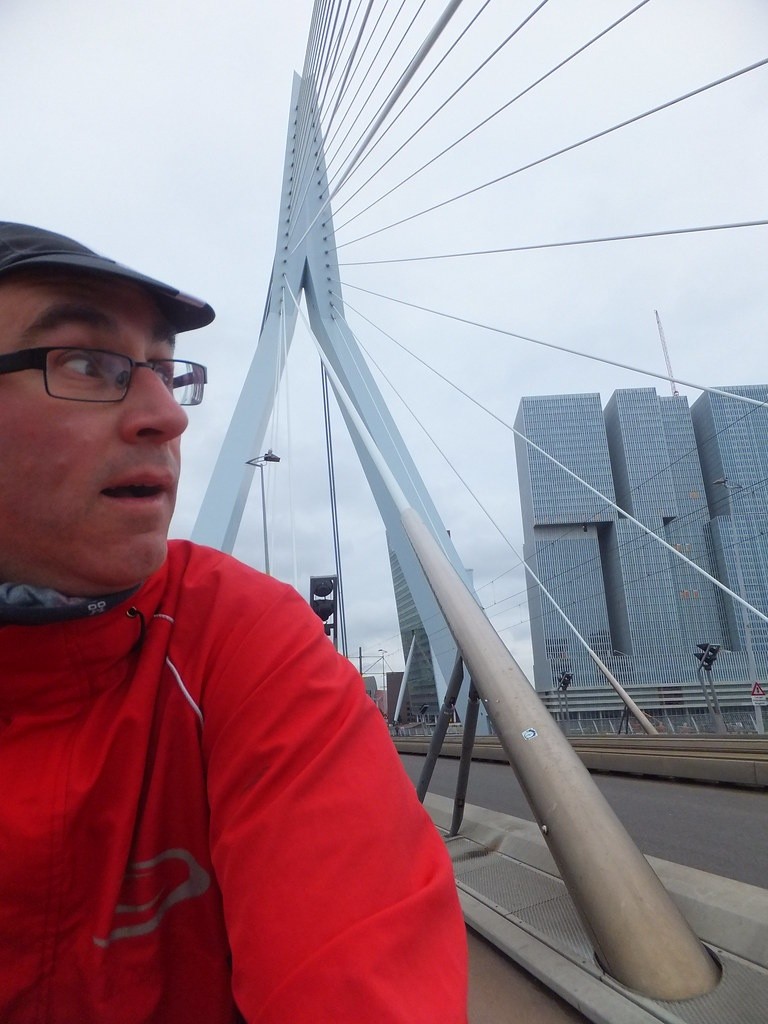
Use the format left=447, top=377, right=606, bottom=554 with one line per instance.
left=245, top=449, right=279, bottom=573
left=557, top=671, right=575, bottom=733
left=378, top=649, right=387, bottom=713
left=694, top=642, right=728, bottom=737
left=419, top=704, right=430, bottom=735
left=308, top=577, right=339, bottom=654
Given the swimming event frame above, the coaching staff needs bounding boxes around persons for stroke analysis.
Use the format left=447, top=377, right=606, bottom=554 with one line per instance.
left=0, top=220, right=468, bottom=1024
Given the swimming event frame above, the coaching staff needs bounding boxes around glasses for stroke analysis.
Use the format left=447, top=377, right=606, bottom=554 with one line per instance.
left=0, top=345, right=208, bottom=406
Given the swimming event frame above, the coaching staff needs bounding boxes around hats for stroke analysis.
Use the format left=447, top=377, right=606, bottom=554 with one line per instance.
left=0, top=220, right=216, bottom=334
